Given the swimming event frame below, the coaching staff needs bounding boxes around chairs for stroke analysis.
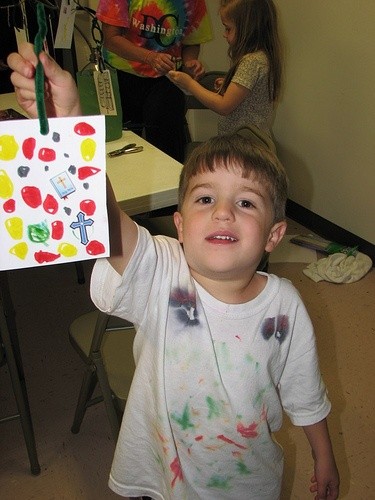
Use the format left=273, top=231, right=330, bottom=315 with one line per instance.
left=67, top=296, right=139, bottom=450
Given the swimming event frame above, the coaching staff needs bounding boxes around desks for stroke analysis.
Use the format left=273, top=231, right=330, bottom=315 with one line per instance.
left=1, top=89, right=188, bottom=283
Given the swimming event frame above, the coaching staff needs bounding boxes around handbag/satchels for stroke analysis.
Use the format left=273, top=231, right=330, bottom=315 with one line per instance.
left=76, top=55, right=123, bottom=142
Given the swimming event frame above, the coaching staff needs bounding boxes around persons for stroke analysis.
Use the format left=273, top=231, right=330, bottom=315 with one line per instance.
left=96, top=0, right=215, bottom=163
left=165, top=1, right=283, bottom=273
left=7, top=42, right=340, bottom=500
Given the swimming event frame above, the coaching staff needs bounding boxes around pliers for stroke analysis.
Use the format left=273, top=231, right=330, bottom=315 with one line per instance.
left=107, top=143, right=143, bottom=158
